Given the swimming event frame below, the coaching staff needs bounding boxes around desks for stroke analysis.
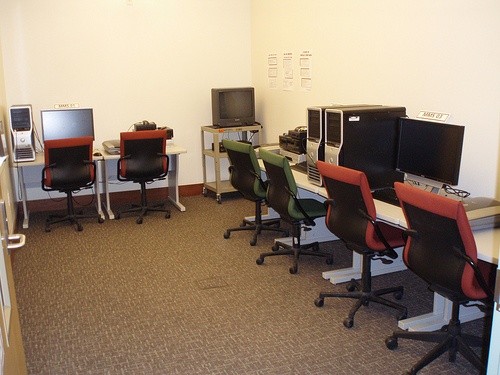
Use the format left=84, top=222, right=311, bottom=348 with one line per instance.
left=252, top=143, right=500, bottom=375
left=98, top=147, right=187, bottom=219
left=11, top=142, right=104, bottom=228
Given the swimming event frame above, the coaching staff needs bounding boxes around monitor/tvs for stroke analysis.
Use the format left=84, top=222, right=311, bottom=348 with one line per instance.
left=395, top=117, right=465, bottom=185
left=211, top=87, right=255, bottom=128
left=40, top=108, right=95, bottom=143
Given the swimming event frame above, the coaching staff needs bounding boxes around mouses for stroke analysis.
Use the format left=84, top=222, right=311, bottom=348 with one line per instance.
left=94, top=152, right=102, bottom=156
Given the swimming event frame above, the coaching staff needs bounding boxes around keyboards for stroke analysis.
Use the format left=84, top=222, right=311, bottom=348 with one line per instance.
left=371, top=188, right=401, bottom=207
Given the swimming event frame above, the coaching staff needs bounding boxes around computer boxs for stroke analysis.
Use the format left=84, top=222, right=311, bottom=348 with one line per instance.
left=307, top=104, right=406, bottom=190
left=9, top=105, right=36, bottom=162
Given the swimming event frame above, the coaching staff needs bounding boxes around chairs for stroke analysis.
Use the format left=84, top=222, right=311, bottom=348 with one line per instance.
left=315, top=161, right=409, bottom=328
left=222, top=138, right=289, bottom=245
left=41, top=136, right=105, bottom=232
left=385, top=182, right=494, bottom=375
left=254, top=146, right=333, bottom=275
left=115, top=130, right=173, bottom=224
